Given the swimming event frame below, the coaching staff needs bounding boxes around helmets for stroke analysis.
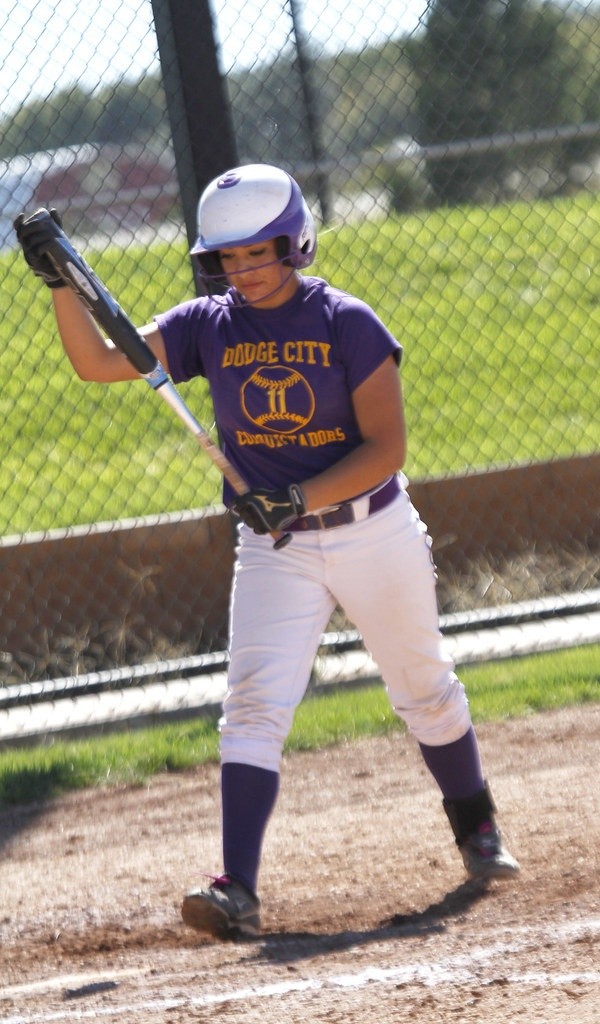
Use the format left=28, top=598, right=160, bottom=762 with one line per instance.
left=190, top=164, right=317, bottom=307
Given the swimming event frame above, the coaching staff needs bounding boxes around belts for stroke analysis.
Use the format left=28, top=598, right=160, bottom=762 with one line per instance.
left=282, top=473, right=401, bottom=532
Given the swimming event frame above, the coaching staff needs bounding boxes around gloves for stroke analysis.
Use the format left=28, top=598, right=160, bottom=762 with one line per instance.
left=13, top=208, right=67, bottom=288
left=234, top=483, right=306, bottom=535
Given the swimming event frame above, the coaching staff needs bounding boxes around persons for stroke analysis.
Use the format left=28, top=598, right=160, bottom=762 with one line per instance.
left=14, top=164, right=523, bottom=941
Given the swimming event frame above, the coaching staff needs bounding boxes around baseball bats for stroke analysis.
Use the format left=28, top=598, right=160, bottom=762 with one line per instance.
left=21, top=207, right=294, bottom=551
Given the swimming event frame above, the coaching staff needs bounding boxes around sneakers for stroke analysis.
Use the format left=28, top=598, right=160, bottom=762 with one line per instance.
left=181, top=874, right=261, bottom=938
left=459, top=825, right=521, bottom=880
